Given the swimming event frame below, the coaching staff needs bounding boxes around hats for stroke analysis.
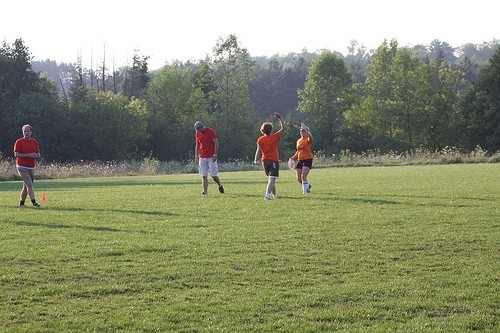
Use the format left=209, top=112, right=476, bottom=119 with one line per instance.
left=194, top=120, right=205, bottom=132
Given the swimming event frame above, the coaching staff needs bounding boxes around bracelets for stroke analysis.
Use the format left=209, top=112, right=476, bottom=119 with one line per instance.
left=213, top=155, right=217, bottom=157
left=254, top=161, right=258, bottom=164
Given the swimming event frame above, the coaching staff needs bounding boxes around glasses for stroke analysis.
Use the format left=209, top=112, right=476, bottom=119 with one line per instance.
left=24, top=130, right=30, bottom=132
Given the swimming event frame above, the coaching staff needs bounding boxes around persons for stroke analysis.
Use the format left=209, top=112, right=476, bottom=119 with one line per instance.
left=253, top=112, right=287, bottom=201
left=13, top=124, right=42, bottom=209
left=194, top=121, right=225, bottom=195
left=290, top=122, right=314, bottom=195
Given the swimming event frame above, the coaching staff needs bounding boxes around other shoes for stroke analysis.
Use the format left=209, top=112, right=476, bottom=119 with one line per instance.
left=33, top=202, right=40, bottom=208
left=218, top=185, right=224, bottom=193
